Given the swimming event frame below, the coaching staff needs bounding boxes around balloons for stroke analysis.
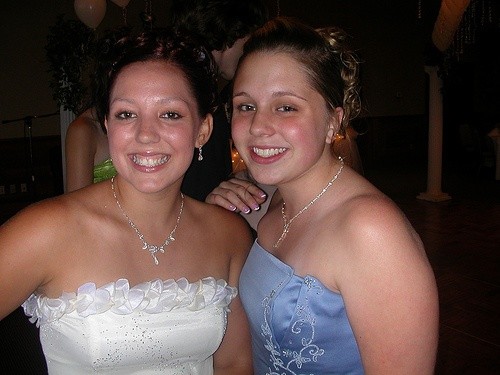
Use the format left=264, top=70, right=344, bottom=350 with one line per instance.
left=73, top=0, right=109, bottom=29
left=112, top=0, right=132, bottom=8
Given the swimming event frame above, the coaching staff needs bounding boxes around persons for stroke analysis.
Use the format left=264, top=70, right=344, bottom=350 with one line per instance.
left=0, top=31, right=255, bottom=375
left=171, top=1, right=266, bottom=81
left=203, top=16, right=440, bottom=375
left=66, top=104, right=123, bottom=195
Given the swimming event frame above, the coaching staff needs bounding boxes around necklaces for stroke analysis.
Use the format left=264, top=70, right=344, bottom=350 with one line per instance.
left=274, top=154, right=345, bottom=248
left=110, top=175, right=185, bottom=266
